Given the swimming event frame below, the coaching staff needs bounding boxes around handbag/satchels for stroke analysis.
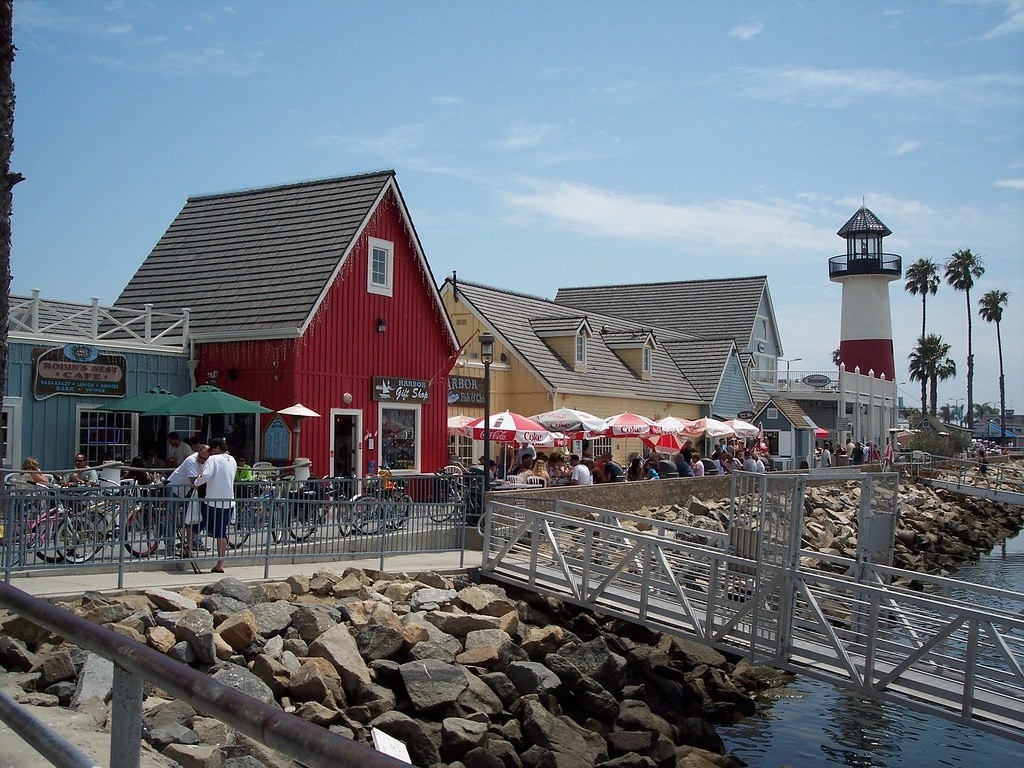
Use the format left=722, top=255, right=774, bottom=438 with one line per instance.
left=185, top=489, right=203, bottom=525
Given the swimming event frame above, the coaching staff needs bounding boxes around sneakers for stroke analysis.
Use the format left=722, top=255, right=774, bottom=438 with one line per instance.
left=191, top=539, right=210, bottom=551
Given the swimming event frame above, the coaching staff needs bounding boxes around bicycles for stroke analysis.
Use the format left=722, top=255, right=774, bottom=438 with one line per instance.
left=0, top=476, right=415, bottom=563
left=428, top=468, right=482, bottom=526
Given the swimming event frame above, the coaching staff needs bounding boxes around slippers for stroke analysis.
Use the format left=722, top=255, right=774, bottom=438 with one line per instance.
left=177, top=551, right=193, bottom=558
left=211, top=567, right=225, bottom=573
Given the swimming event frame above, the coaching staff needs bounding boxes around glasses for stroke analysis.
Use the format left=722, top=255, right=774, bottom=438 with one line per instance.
left=238, top=458, right=246, bottom=463
left=198, top=455, right=207, bottom=461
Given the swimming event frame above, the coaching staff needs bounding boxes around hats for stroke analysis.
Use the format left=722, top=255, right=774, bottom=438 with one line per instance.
left=207, top=437, right=224, bottom=453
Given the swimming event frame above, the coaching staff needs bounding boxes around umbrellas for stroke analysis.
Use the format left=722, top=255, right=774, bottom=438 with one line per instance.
left=749, top=421, right=769, bottom=457
left=91, top=380, right=274, bottom=418
left=723, top=418, right=759, bottom=448
left=680, top=415, right=734, bottom=460
left=813, top=427, right=829, bottom=438
left=455, top=408, right=572, bottom=482
left=638, top=432, right=701, bottom=457
left=605, top=411, right=665, bottom=469
left=884, top=435, right=895, bottom=471
left=652, top=415, right=706, bottom=460
left=525, top=406, right=610, bottom=459
left=445, top=413, right=477, bottom=437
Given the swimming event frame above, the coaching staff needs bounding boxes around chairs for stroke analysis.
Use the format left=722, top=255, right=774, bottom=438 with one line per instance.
left=527, top=476, right=547, bottom=488
left=506, top=475, right=523, bottom=484
left=252, top=462, right=279, bottom=480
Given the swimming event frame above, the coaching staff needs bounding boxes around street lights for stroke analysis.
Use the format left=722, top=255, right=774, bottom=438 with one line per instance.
left=778, top=358, right=802, bottom=393
left=478, top=331, right=496, bottom=495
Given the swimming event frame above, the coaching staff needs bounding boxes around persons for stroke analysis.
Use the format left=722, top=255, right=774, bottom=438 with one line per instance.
left=812, top=437, right=872, bottom=468
left=20, top=429, right=252, bottom=573
left=989, top=439, right=1014, bottom=455
left=970, top=450, right=991, bottom=488
left=479, top=430, right=779, bottom=488
